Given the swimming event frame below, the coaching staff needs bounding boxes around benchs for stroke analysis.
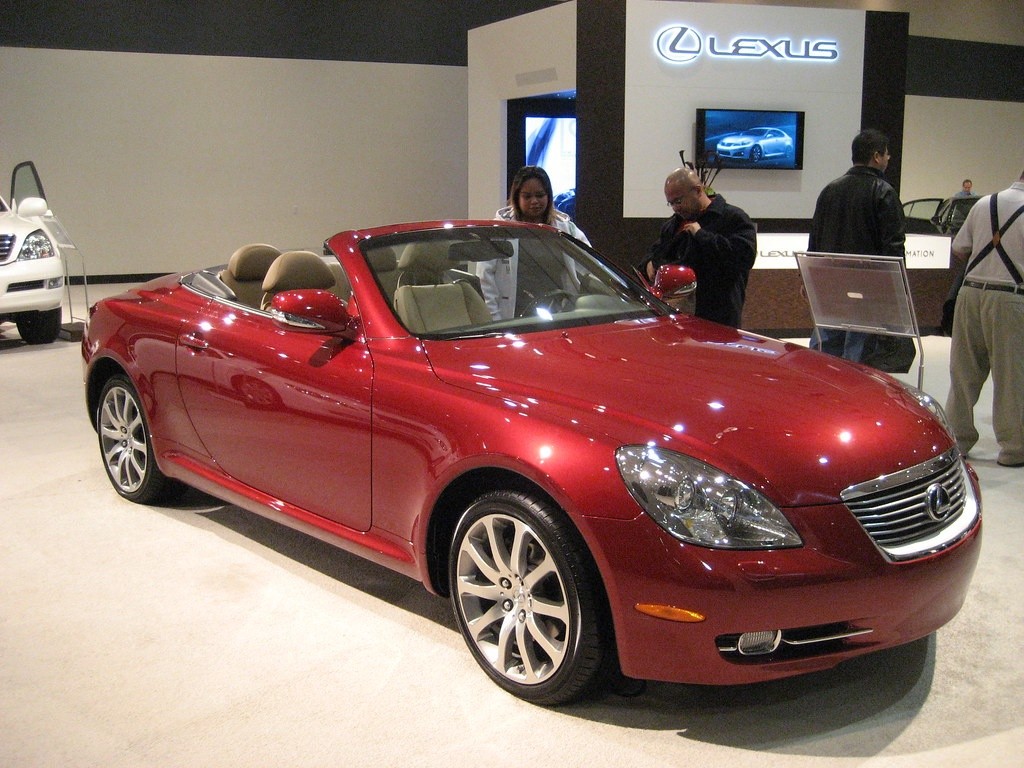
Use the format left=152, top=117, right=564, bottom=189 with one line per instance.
left=216, top=243, right=399, bottom=311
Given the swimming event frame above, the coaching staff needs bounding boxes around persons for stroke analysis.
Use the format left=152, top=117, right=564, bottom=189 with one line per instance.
left=476, top=165, right=594, bottom=321
left=807, top=129, right=908, bottom=367
left=945, top=170, right=1024, bottom=468
left=640, top=166, right=757, bottom=329
left=955, top=179, right=978, bottom=198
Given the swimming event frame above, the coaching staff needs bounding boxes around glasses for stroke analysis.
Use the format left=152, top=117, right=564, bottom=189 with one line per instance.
left=667, top=187, right=696, bottom=207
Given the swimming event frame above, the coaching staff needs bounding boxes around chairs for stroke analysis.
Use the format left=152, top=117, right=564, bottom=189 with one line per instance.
left=392, top=240, right=494, bottom=333
left=260, top=250, right=337, bottom=314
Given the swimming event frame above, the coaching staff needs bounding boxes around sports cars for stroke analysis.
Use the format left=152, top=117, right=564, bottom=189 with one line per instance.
left=82, top=220, right=984, bottom=707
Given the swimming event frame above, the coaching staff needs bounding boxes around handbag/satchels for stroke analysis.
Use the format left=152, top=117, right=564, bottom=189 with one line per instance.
left=859, top=333, right=916, bottom=374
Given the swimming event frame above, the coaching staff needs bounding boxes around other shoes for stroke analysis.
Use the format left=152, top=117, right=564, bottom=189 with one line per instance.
left=997, top=460, right=1024, bottom=467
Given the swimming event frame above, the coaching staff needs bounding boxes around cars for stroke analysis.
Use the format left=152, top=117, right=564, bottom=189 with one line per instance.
left=0, top=161, right=68, bottom=343
left=903, top=196, right=982, bottom=237
left=716, top=127, right=794, bottom=163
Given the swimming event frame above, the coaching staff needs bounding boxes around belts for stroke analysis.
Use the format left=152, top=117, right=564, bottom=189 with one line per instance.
left=963, top=280, right=1024, bottom=296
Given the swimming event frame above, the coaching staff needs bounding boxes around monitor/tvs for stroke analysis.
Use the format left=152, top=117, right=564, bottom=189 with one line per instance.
left=696, top=107, right=805, bottom=169
